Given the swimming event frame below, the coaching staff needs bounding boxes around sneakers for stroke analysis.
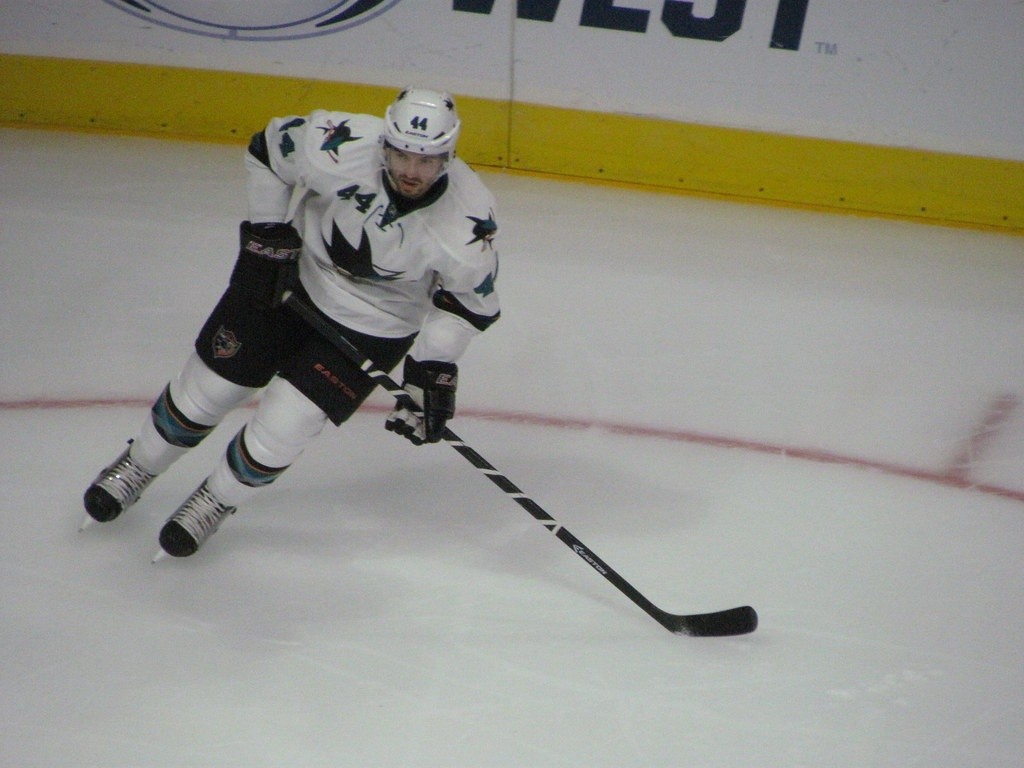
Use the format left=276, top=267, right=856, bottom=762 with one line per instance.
left=78, top=439, right=157, bottom=532
left=151, top=476, right=236, bottom=564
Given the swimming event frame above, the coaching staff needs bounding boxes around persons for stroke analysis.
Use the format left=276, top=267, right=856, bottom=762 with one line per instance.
left=80, top=88, right=502, bottom=563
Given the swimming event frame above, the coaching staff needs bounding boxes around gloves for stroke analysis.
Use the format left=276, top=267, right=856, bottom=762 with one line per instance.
left=229, top=220, right=303, bottom=315
left=385, top=354, right=459, bottom=447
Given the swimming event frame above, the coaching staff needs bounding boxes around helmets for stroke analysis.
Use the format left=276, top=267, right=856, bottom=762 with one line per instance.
left=382, top=89, right=461, bottom=161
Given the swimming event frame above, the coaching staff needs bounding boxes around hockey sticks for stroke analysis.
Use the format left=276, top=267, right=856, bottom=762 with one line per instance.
left=280, top=290, right=759, bottom=639
